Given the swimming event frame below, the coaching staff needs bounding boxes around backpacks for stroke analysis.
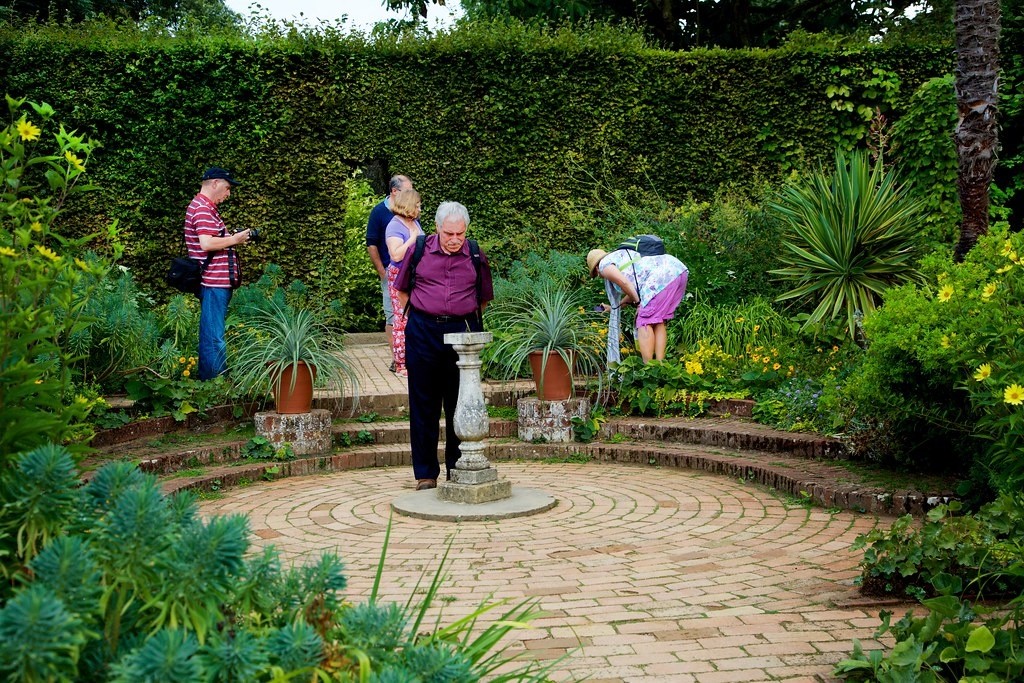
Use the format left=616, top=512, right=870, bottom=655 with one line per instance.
left=615, top=234, right=664, bottom=256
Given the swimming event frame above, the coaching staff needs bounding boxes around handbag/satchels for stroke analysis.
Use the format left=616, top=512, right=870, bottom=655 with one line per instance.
left=166, top=257, right=204, bottom=294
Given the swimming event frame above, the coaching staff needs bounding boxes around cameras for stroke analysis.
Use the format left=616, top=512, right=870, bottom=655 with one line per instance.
left=594, top=305, right=605, bottom=312
left=234, top=227, right=261, bottom=241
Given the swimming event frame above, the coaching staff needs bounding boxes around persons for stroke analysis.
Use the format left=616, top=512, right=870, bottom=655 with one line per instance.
left=183, top=166, right=252, bottom=384
left=365, top=173, right=413, bottom=372
left=586, top=247, right=689, bottom=366
left=392, top=200, right=495, bottom=491
left=384, top=187, right=426, bottom=377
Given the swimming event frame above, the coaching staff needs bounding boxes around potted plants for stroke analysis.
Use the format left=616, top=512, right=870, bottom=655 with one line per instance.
left=212, top=287, right=367, bottom=424
left=480, top=275, right=643, bottom=417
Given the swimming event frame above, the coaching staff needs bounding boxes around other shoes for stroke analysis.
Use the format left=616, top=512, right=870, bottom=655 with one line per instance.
left=396, top=370, right=408, bottom=378
left=416, top=479, right=436, bottom=490
left=390, top=362, right=396, bottom=372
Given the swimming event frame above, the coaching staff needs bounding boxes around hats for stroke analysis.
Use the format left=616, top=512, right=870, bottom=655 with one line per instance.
left=202, top=168, right=243, bottom=188
left=587, top=249, right=609, bottom=279
